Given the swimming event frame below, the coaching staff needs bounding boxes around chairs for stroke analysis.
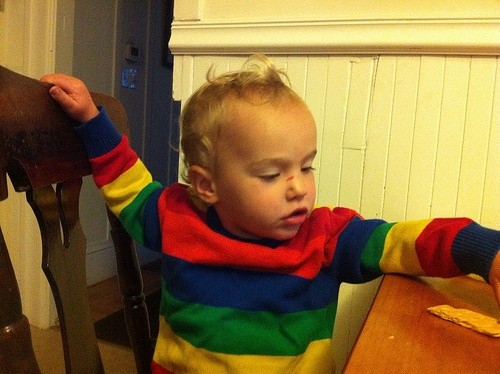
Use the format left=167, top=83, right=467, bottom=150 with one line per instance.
left=0, top=65, right=153, bottom=374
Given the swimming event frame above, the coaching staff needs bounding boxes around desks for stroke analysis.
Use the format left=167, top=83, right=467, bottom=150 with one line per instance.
left=340, top=273, right=500, bottom=374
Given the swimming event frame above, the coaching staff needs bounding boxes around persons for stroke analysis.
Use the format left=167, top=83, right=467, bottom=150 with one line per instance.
left=40, top=54, right=500, bottom=374
